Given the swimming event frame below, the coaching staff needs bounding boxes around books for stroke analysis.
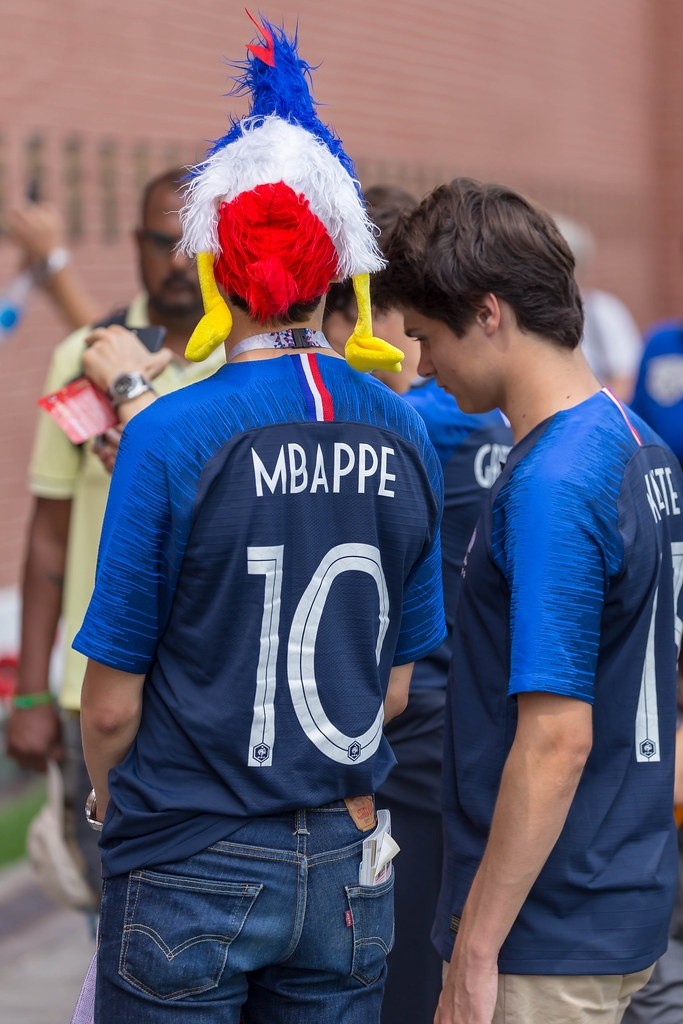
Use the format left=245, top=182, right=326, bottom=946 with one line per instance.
left=360, top=802, right=399, bottom=886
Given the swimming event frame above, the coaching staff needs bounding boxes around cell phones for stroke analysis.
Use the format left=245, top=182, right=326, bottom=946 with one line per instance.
left=125, top=325, right=167, bottom=353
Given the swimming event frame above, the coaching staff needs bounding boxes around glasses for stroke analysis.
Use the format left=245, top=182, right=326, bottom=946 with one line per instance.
left=144, top=229, right=183, bottom=260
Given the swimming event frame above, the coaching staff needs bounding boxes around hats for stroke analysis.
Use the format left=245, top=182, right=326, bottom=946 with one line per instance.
left=182, top=7, right=405, bottom=373
left=26, top=756, right=104, bottom=913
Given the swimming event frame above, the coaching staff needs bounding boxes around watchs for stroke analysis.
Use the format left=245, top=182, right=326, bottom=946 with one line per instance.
left=104, top=368, right=153, bottom=407
left=86, top=789, right=104, bottom=831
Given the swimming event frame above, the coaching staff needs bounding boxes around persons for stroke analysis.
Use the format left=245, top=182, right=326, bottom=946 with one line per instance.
left=3, top=164, right=683, bottom=1024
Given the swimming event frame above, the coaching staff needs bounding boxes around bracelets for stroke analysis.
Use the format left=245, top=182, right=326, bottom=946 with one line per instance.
left=12, top=690, right=51, bottom=712
